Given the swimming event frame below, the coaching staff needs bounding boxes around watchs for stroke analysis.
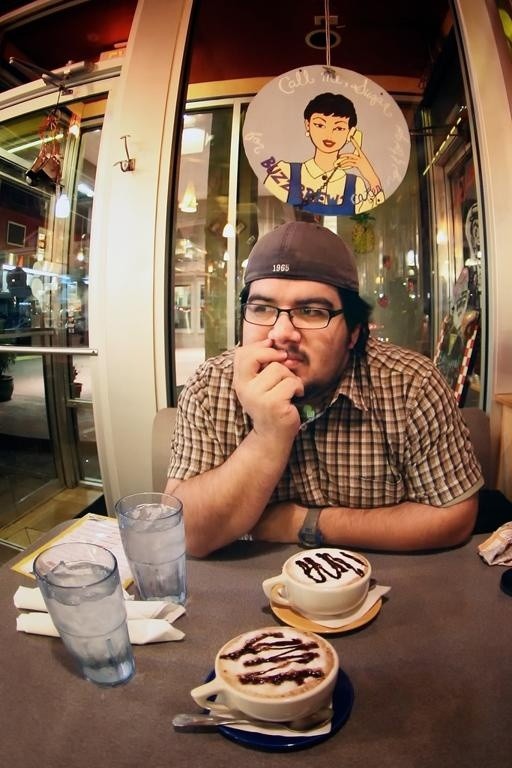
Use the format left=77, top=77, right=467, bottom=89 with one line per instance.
left=299, top=504, right=328, bottom=548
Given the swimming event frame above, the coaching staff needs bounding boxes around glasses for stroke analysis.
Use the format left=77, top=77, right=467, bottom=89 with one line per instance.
left=241, top=291, right=347, bottom=329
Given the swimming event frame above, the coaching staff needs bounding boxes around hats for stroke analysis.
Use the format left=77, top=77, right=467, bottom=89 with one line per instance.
left=245, top=221, right=360, bottom=295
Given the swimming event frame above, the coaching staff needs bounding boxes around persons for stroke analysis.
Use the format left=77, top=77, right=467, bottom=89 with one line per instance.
left=264, top=92, right=384, bottom=217
left=159, top=223, right=485, bottom=561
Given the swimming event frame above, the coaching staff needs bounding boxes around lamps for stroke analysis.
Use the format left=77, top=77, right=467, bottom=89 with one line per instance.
left=178, top=175, right=199, bottom=213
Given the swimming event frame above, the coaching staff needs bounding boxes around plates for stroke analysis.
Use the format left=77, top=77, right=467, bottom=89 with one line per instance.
left=197, top=662, right=356, bottom=750
left=266, top=582, right=383, bottom=636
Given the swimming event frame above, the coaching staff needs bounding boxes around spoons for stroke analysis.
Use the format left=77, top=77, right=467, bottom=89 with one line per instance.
left=169, top=704, right=335, bottom=732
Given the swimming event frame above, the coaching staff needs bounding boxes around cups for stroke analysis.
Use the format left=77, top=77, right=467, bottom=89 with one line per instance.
left=31, top=543, right=137, bottom=685
left=190, top=624, right=342, bottom=724
left=115, top=491, right=190, bottom=615
left=263, top=547, right=374, bottom=620
